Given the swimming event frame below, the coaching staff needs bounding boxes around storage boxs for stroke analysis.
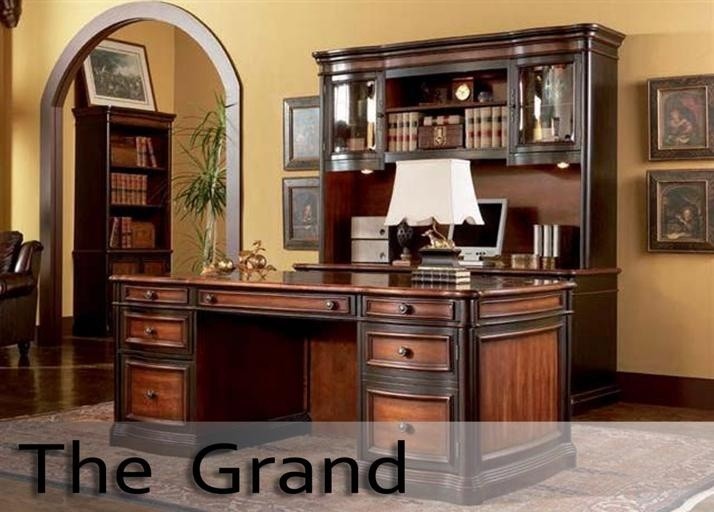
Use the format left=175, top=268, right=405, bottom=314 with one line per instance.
left=351, top=240, right=398, bottom=263
left=418, top=125, right=463, bottom=147
left=351, top=216, right=395, bottom=240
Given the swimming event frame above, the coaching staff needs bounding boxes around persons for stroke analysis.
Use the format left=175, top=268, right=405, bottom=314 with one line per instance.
left=669, top=109, right=694, bottom=146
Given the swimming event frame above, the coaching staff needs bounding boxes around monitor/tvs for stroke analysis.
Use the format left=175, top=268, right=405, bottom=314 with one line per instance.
left=447, top=198, right=510, bottom=268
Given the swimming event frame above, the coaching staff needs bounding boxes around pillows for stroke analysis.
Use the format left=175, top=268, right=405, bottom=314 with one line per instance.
left=0, top=230, right=23, bottom=273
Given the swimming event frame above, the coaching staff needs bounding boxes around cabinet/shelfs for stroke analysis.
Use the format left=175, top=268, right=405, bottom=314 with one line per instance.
left=112, top=271, right=575, bottom=505
left=72, top=108, right=175, bottom=339
left=290, top=23, right=627, bottom=411
left=283, top=96, right=320, bottom=170
left=282, top=176, right=320, bottom=250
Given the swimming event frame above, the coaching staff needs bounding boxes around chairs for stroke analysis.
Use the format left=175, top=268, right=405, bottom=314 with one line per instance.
left=0, top=240, right=44, bottom=367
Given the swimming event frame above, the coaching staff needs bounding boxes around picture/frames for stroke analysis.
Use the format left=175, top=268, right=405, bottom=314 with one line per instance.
left=647, top=74, right=714, bottom=161
left=646, top=168, right=714, bottom=253
left=81, top=38, right=157, bottom=111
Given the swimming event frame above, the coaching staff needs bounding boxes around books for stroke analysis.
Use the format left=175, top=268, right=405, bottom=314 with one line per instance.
left=109, top=133, right=160, bottom=249
left=386, top=112, right=425, bottom=150
left=422, top=115, right=463, bottom=125
left=463, top=106, right=507, bottom=149
left=533, top=223, right=579, bottom=258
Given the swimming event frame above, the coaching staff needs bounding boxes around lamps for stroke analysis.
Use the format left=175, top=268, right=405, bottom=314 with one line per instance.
left=384, top=159, right=485, bottom=282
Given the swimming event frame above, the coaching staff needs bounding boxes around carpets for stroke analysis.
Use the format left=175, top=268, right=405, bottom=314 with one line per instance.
left=0, top=403, right=714, bottom=512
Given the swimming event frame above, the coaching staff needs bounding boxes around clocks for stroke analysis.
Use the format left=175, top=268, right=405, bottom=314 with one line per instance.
left=452, top=77, right=474, bottom=103
